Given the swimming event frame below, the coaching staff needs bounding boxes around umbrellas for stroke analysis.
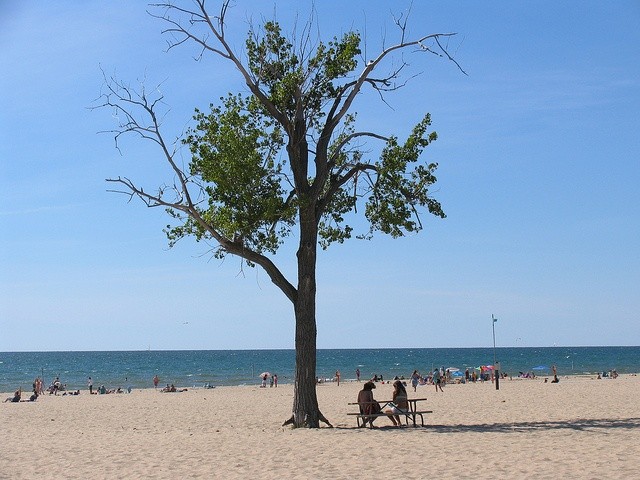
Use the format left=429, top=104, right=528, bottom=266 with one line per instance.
left=258, top=372, right=271, bottom=377
left=477, top=366, right=493, bottom=372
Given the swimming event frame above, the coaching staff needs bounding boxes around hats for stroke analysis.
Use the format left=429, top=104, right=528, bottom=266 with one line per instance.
left=367, top=382, right=376, bottom=388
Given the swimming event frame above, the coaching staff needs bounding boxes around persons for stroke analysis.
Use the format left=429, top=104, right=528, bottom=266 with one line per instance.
left=32, top=379, right=42, bottom=392
left=552, top=362, right=558, bottom=374
left=394, top=375, right=398, bottom=379
left=269, top=376, right=273, bottom=386
left=382, top=381, right=385, bottom=383
left=153, top=375, right=159, bottom=388
left=357, top=382, right=379, bottom=428
left=54, top=379, right=60, bottom=395
left=171, top=386, right=174, bottom=390
left=370, top=375, right=377, bottom=382
left=261, top=374, right=268, bottom=387
left=596, top=371, right=601, bottom=379
left=503, top=372, right=507, bottom=375
left=77, top=389, right=80, bottom=394
left=472, top=372, right=475, bottom=381
left=88, top=377, right=92, bottom=393
left=480, top=366, right=484, bottom=382
left=96, top=386, right=100, bottom=392
left=3, top=387, right=21, bottom=404
left=27, top=394, right=38, bottom=402
left=125, top=377, right=127, bottom=380
left=424, top=375, right=432, bottom=383
left=465, top=368, right=470, bottom=383
left=409, top=371, right=424, bottom=391
left=263, top=375, right=266, bottom=386
left=127, top=386, right=131, bottom=393
left=600, top=370, right=608, bottom=377
left=549, top=374, right=558, bottom=383
left=387, top=381, right=391, bottom=385
left=101, top=385, right=106, bottom=394
left=439, top=367, right=442, bottom=378
left=517, top=372, right=531, bottom=377
left=446, top=370, right=451, bottom=382
left=356, top=369, right=361, bottom=382
left=335, top=368, right=340, bottom=385
left=430, top=368, right=443, bottom=393
left=117, top=388, right=122, bottom=392
left=274, top=375, right=278, bottom=387
left=385, top=380, right=408, bottom=427
left=169, top=384, right=176, bottom=392
left=73, top=391, right=76, bottom=395
left=159, top=384, right=169, bottom=392
left=612, top=370, right=617, bottom=377
left=400, top=376, right=404, bottom=379
left=50, top=376, right=58, bottom=396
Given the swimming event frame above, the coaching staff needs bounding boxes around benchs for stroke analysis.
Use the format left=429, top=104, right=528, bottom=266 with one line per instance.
left=347, top=398, right=433, bottom=427
